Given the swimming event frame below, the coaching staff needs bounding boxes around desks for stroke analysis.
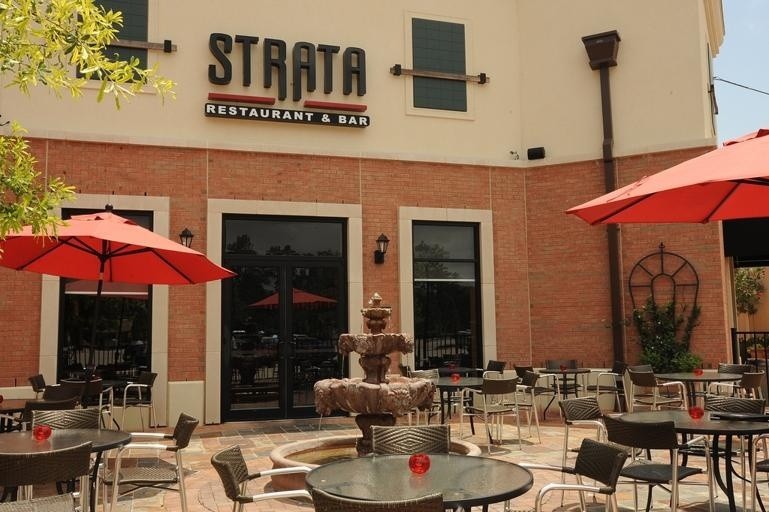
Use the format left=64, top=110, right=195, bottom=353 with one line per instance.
left=652, top=369, right=744, bottom=407
left=433, top=376, right=484, bottom=435
left=302, top=452, right=534, bottom=512
left=539, top=366, right=591, bottom=400
left=617, top=409, right=769, bottom=511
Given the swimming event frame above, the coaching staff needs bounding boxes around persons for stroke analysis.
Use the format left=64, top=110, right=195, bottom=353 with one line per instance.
left=60, top=280, right=151, bottom=364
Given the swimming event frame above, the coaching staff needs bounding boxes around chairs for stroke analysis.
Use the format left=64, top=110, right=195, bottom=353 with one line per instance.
left=210, top=442, right=314, bottom=512
left=555, top=395, right=646, bottom=508
left=231, top=323, right=341, bottom=410
left=396, top=360, right=564, bottom=456
left=749, top=432, right=769, bottom=512
left=413, top=353, right=471, bottom=370
left=368, top=422, right=451, bottom=455
left=601, top=412, right=716, bottom=511
left=500, top=438, right=629, bottom=512
left=699, top=393, right=767, bottom=415
left=1, top=368, right=200, bottom=510
left=585, top=360, right=767, bottom=415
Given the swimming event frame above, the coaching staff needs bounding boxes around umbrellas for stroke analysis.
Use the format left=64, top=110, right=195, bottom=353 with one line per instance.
left=563, top=125, right=769, bottom=226
left=1, top=202, right=237, bottom=409
left=248, top=288, right=340, bottom=334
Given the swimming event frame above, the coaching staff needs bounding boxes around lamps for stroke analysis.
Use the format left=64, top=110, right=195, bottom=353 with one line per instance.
left=178, top=227, right=196, bottom=249
left=373, top=230, right=392, bottom=265
